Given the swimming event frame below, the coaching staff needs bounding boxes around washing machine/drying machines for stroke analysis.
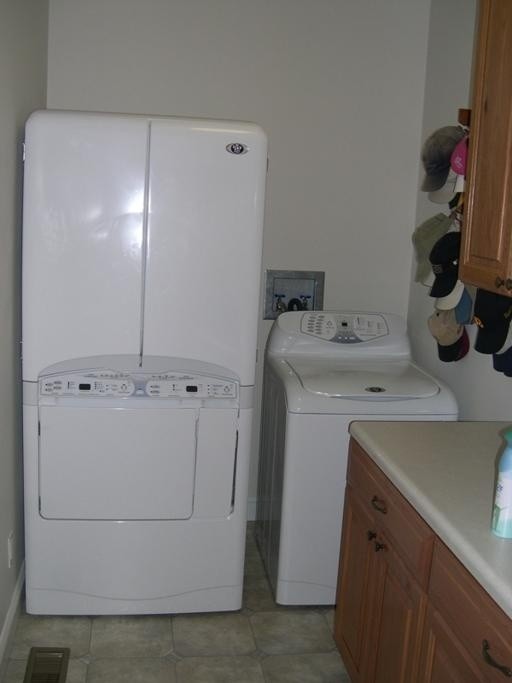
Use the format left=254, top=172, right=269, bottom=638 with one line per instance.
left=255, top=310, right=458, bottom=606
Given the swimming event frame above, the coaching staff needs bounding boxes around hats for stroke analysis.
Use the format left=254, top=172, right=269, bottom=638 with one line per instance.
left=411, top=125, right=512, bottom=378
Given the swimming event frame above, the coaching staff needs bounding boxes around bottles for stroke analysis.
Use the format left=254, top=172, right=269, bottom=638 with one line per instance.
left=488, top=427, right=512, bottom=541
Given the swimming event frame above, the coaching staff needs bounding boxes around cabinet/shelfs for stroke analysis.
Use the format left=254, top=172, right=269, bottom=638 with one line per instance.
left=459, top=0, right=512, bottom=294
left=329, top=437, right=511, bottom=683
